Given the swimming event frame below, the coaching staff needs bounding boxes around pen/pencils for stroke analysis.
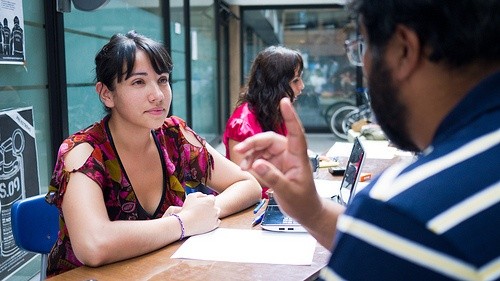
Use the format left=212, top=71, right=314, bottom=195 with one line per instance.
left=253, top=198, right=266, bottom=213
left=250, top=213, right=264, bottom=226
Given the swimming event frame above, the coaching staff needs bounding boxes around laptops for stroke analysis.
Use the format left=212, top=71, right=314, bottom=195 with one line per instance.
left=260, top=137, right=366, bottom=233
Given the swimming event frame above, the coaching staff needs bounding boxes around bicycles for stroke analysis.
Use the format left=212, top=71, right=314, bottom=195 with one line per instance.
left=325, top=89, right=371, bottom=140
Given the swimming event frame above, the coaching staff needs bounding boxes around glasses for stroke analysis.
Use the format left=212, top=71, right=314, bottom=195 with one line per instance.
left=344, top=35, right=368, bottom=65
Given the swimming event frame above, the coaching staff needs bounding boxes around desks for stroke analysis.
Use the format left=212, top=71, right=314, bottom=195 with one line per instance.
left=41, top=156, right=392, bottom=281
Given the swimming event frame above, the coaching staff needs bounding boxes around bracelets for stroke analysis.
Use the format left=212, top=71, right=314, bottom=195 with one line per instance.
left=169, top=213, right=185, bottom=240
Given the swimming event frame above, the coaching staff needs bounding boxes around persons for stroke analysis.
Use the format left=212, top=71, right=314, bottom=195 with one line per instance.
left=222, top=46, right=305, bottom=198
left=45, top=29, right=264, bottom=277
left=232, top=0, right=500, bottom=281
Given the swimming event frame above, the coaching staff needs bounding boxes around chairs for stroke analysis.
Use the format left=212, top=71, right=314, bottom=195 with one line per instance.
left=11, top=193, right=61, bottom=281
